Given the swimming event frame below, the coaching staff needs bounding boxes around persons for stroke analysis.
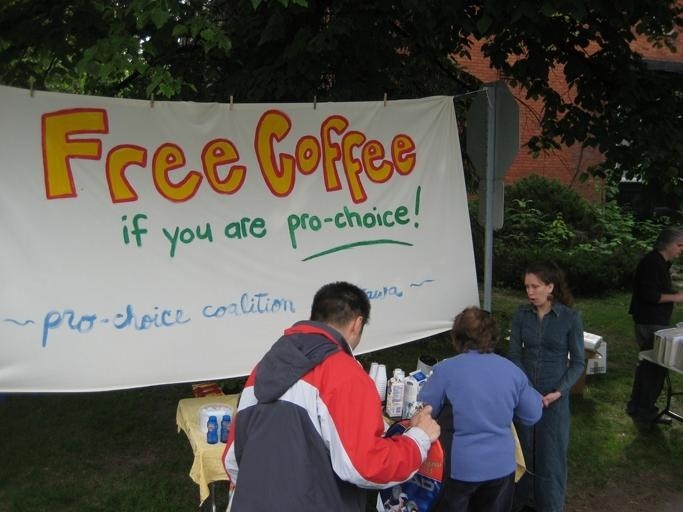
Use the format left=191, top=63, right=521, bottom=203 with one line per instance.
left=506, top=256, right=587, bottom=510
left=218, top=280, right=442, bottom=510
left=624, top=227, right=683, bottom=424
left=410, top=305, right=544, bottom=511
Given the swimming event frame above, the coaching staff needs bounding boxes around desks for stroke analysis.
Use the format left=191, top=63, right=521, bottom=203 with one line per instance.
left=177, top=393, right=527, bottom=507
left=639, top=348, right=683, bottom=428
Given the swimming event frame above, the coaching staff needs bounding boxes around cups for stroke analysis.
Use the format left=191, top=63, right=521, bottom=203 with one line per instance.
left=368, top=362, right=387, bottom=402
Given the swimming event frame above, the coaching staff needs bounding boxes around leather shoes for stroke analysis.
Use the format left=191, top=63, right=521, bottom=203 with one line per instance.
left=626, top=403, right=635, bottom=413
left=636, top=411, right=672, bottom=423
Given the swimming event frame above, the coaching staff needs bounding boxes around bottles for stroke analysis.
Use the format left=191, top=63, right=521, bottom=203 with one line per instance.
left=220, top=415, right=231, bottom=443
left=206, top=416, right=218, bottom=444
